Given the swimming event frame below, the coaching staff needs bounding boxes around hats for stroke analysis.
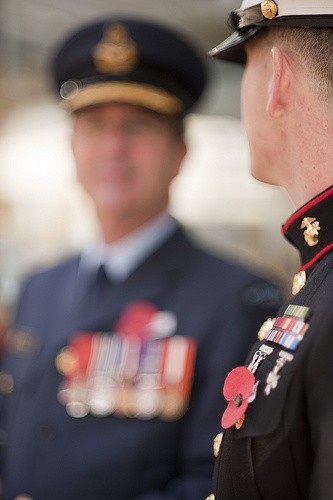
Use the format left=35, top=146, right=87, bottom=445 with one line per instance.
left=49, top=17, right=210, bottom=121
left=208, top=0, right=332, bottom=65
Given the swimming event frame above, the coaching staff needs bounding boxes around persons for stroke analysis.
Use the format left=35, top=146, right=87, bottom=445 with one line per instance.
left=1, top=14, right=281, bottom=500
left=172, top=0, right=332, bottom=500
